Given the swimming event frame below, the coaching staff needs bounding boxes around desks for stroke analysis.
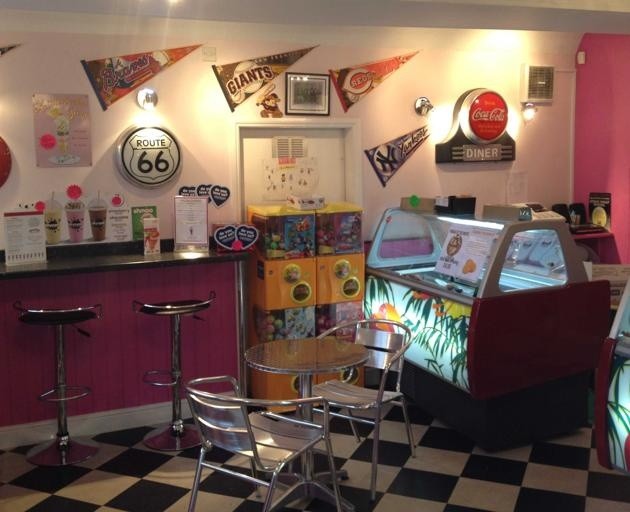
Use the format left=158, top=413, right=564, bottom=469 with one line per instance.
left=242, top=336, right=372, bottom=512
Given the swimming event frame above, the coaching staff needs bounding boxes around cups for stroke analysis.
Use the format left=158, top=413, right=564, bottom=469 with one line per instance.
left=87, top=197, right=110, bottom=242
left=62, top=198, right=88, bottom=242
left=43, top=200, right=64, bottom=246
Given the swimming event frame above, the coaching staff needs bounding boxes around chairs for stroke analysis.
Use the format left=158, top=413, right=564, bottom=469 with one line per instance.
left=302, top=318, right=414, bottom=504
left=183, top=372, right=350, bottom=512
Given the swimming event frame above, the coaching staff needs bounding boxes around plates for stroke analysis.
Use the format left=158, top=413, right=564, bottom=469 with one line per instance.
left=592, top=206, right=607, bottom=228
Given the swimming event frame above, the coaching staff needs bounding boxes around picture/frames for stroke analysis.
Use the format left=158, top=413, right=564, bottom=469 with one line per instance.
left=285, top=71, right=331, bottom=117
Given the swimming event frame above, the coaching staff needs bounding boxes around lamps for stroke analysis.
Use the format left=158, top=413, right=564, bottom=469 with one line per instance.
left=414, top=96, right=434, bottom=116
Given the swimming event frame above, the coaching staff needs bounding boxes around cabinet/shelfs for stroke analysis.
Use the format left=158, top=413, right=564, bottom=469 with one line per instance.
left=0, top=245, right=253, bottom=464
left=360, top=204, right=616, bottom=454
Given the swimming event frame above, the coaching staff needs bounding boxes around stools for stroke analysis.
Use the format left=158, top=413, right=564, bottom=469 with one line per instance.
left=131, top=291, right=227, bottom=454
left=589, top=334, right=622, bottom=472
left=10, top=304, right=105, bottom=468
